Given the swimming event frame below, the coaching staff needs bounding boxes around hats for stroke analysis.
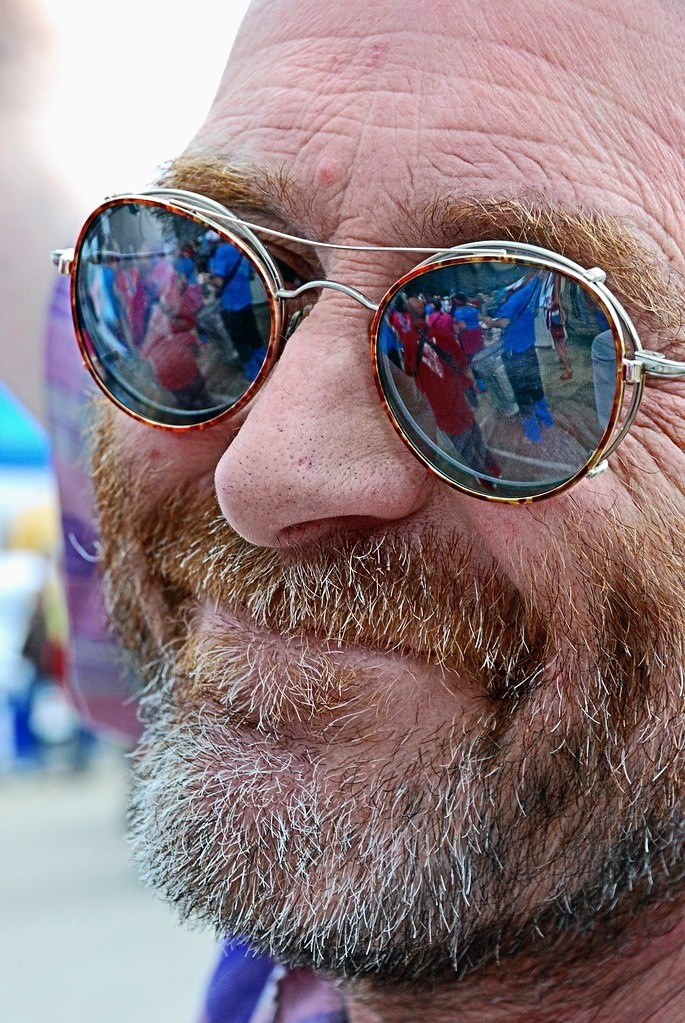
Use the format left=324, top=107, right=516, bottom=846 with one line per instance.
left=197, top=229, right=220, bottom=242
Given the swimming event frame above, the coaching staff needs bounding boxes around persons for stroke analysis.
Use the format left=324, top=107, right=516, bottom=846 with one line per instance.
left=388, top=270, right=593, bottom=490
left=86, top=230, right=267, bottom=412
left=85, top=1, right=683, bottom=1023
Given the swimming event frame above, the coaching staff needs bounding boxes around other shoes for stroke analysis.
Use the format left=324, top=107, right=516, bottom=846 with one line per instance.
left=474, top=379, right=487, bottom=394
left=484, top=461, right=503, bottom=479
left=505, top=404, right=520, bottom=418
left=475, top=476, right=497, bottom=491
left=559, top=371, right=573, bottom=379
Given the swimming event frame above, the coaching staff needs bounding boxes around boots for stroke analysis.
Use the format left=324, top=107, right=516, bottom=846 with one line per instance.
left=534, top=399, right=556, bottom=429
left=517, top=417, right=541, bottom=443
left=243, top=360, right=261, bottom=380
left=252, top=346, right=267, bottom=362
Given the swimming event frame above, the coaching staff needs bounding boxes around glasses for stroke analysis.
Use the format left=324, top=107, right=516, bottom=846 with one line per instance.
left=52, top=187, right=685, bottom=502
